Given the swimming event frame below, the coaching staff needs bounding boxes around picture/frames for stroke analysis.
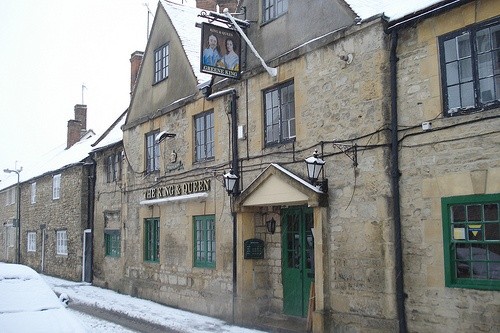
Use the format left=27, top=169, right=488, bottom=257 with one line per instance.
left=200, top=22, right=241, bottom=79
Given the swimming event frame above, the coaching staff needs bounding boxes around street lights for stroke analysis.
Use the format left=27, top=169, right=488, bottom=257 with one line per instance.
left=3, top=169, right=21, bottom=264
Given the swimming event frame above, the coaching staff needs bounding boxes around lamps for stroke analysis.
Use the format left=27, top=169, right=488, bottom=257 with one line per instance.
left=265, top=217, right=276, bottom=234
left=223, top=169, right=241, bottom=198
left=304, top=149, right=328, bottom=192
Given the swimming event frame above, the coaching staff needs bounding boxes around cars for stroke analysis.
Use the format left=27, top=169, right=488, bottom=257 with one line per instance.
left=457, top=247, right=500, bottom=280
